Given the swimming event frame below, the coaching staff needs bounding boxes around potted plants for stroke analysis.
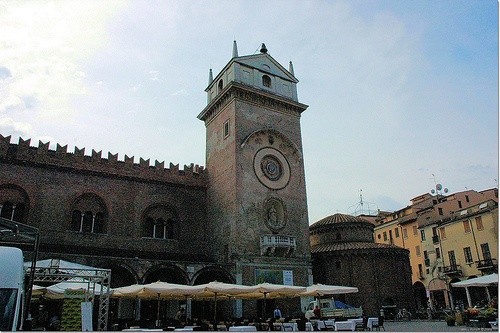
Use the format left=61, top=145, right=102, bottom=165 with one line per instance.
left=446, top=306, right=497, bottom=328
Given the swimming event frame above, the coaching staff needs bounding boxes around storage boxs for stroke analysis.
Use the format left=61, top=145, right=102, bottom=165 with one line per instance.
left=60, top=289, right=87, bottom=331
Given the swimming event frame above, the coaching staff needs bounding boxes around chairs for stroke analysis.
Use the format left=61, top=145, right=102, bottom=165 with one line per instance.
left=110, top=316, right=386, bottom=331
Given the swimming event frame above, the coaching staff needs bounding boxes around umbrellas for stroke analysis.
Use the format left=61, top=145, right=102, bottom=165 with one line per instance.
left=298, top=284, right=358, bottom=318
left=193, top=280, right=257, bottom=317
left=30, top=280, right=196, bottom=324
left=450, top=272, right=498, bottom=308
left=239, top=282, right=306, bottom=319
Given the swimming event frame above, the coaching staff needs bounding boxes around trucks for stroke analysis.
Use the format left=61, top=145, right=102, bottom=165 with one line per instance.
left=302, top=297, right=363, bottom=320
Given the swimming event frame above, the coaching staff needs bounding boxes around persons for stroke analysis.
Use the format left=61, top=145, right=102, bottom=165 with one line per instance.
left=274, top=306, right=282, bottom=319
left=313, top=305, right=322, bottom=319
